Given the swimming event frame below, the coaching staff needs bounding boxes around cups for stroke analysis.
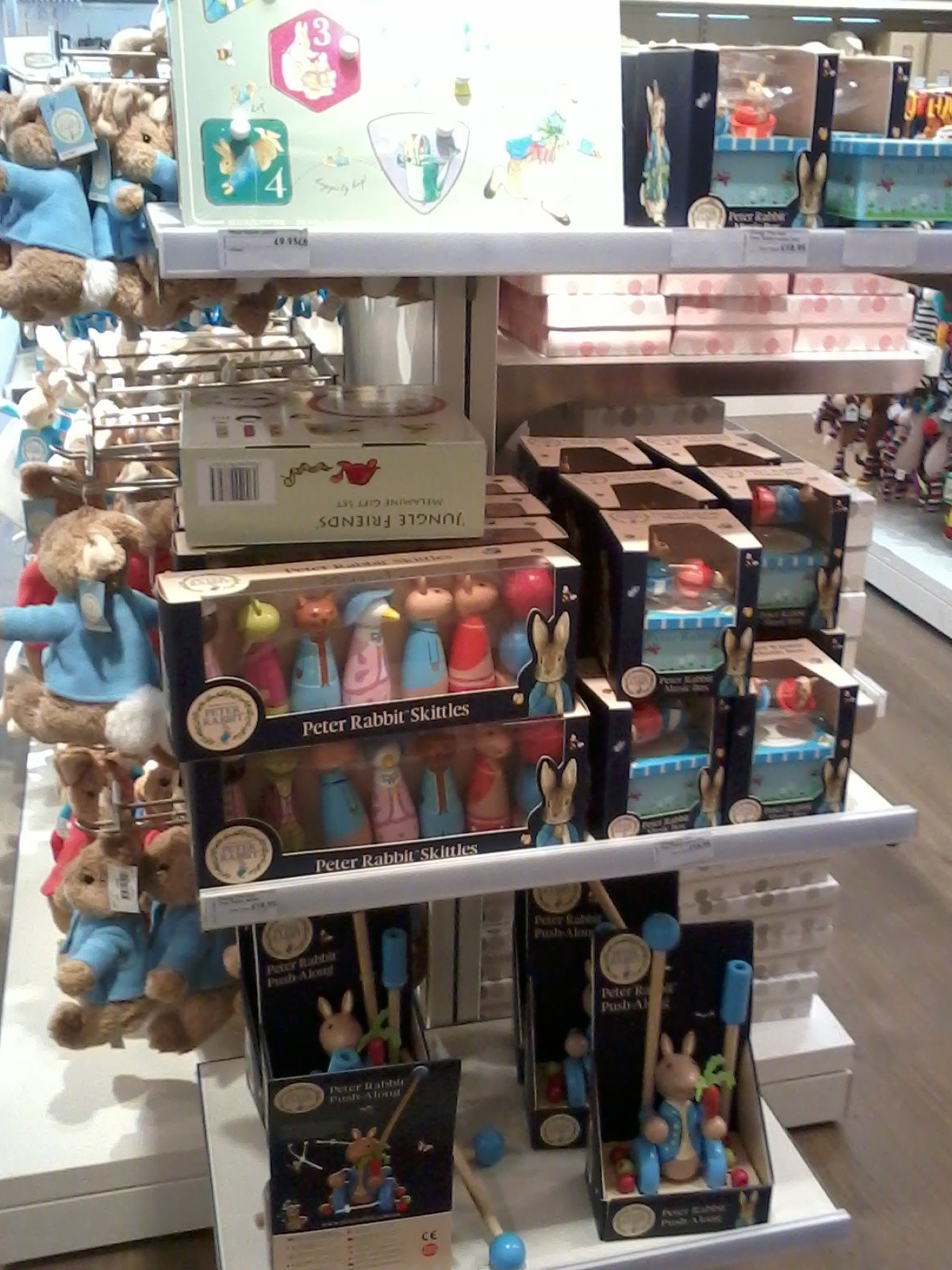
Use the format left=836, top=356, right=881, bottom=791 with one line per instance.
left=342, top=289, right=436, bottom=415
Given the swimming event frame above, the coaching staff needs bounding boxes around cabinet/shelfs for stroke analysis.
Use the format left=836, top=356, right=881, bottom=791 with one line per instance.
left=143, top=198, right=952, bottom=1270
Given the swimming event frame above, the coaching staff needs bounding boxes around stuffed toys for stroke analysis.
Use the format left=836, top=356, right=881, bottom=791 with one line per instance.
left=814, top=382, right=951, bottom=542
left=0, top=5, right=247, bottom=1054
